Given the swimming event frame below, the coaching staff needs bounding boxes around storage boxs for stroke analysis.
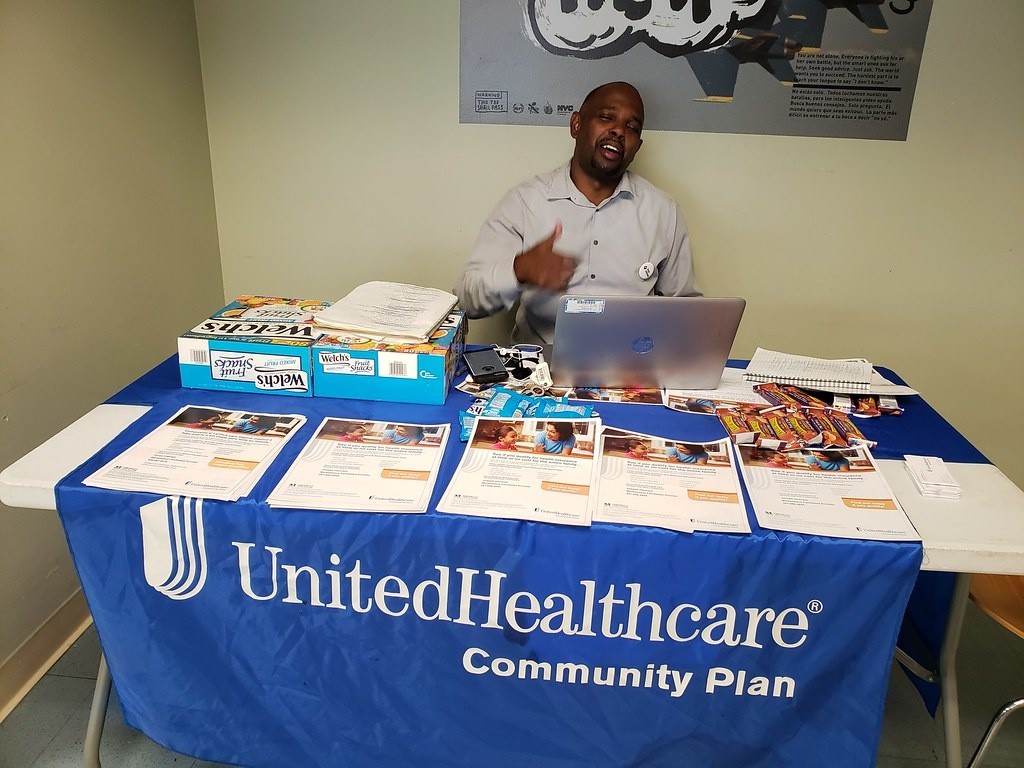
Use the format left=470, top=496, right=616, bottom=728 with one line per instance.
left=175, top=292, right=334, bottom=400
left=312, top=306, right=472, bottom=403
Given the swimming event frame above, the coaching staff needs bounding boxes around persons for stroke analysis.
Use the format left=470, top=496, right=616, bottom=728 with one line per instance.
left=381, top=424, right=425, bottom=447
left=625, top=440, right=653, bottom=462
left=184, top=413, right=222, bottom=431
left=666, top=443, right=709, bottom=466
left=761, top=450, right=796, bottom=470
left=805, top=450, right=851, bottom=473
left=455, top=82, right=708, bottom=349
left=490, top=425, right=519, bottom=452
left=231, top=414, right=269, bottom=436
left=335, top=425, right=367, bottom=444
left=532, top=421, right=577, bottom=456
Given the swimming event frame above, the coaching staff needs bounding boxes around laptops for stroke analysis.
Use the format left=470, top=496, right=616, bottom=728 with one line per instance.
left=538, top=296, right=745, bottom=389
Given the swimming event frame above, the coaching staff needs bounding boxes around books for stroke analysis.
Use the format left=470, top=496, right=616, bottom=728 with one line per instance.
left=742, top=346, right=873, bottom=390
left=312, top=280, right=459, bottom=344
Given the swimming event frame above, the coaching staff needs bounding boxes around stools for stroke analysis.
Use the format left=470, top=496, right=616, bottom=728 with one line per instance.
left=965, top=572, right=1024, bottom=768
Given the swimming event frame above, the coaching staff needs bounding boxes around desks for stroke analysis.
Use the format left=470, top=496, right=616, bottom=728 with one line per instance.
left=0, top=350, right=1024, bottom=767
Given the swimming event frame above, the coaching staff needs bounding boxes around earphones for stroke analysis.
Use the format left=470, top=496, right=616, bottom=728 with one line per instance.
left=499, top=347, right=516, bottom=355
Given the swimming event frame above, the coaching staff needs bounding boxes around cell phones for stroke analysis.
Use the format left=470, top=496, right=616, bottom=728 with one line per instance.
left=461, top=347, right=509, bottom=384
